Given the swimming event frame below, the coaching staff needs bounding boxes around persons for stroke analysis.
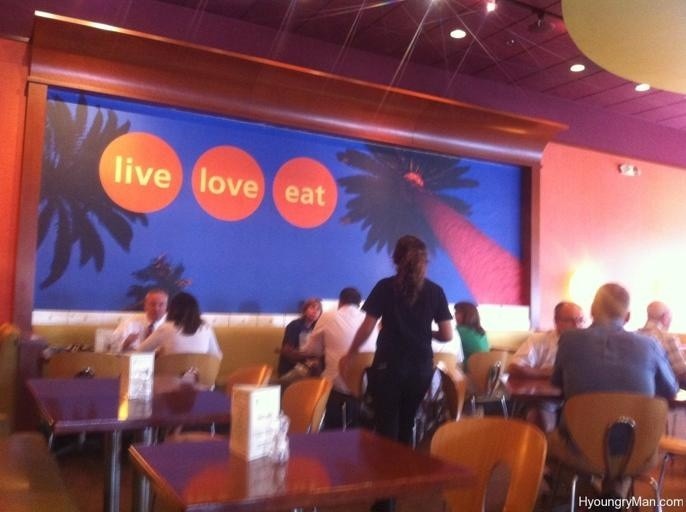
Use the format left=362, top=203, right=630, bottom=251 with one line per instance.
left=541, top=283, right=681, bottom=512
left=299, top=287, right=381, bottom=432
left=453, top=302, right=490, bottom=377
left=507, top=302, right=586, bottom=432
left=133, top=291, right=224, bottom=393
left=277, top=297, right=325, bottom=392
left=348, top=235, right=453, bottom=512
left=110, top=289, right=169, bottom=353
left=431, top=317, right=463, bottom=419
left=634, top=301, right=686, bottom=387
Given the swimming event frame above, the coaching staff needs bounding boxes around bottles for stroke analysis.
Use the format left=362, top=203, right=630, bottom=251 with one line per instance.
left=266, top=465, right=289, bottom=496
left=268, top=408, right=291, bottom=464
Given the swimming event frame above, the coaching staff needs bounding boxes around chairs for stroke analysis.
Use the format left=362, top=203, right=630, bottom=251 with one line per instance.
left=0, top=327, right=686, bottom=512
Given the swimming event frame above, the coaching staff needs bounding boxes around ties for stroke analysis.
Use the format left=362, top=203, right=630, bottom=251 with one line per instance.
left=144, top=323, right=155, bottom=340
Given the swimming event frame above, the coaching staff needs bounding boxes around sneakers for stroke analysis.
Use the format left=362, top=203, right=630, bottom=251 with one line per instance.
left=584, top=490, right=627, bottom=512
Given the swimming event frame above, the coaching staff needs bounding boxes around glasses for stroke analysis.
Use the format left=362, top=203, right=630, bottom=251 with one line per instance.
left=558, top=316, right=588, bottom=328
